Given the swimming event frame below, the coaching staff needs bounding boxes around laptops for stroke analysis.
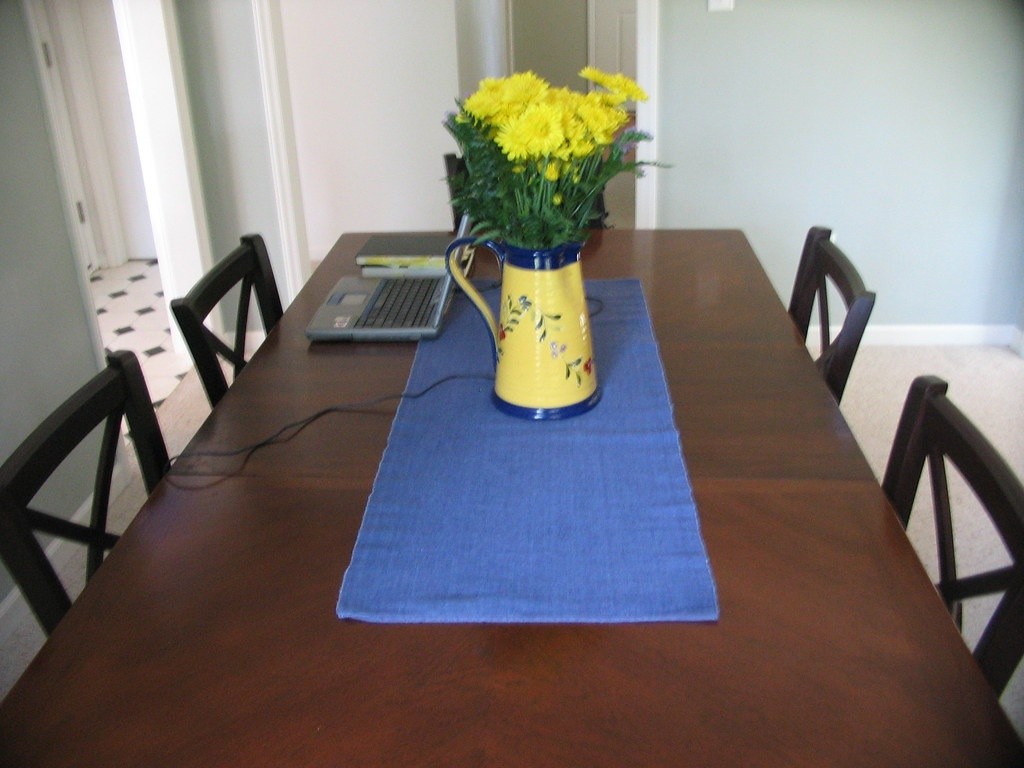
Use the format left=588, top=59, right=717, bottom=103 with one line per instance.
left=304, top=192, right=477, bottom=344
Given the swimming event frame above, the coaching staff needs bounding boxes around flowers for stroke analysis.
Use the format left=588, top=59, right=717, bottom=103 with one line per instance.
left=443, top=65, right=674, bottom=252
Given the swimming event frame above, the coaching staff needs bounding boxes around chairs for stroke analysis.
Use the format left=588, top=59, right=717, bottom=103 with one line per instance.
left=787, top=225, right=875, bottom=408
left=1, top=349, right=172, bottom=639
left=881, top=374, right=1023, bottom=701
left=171, top=234, right=284, bottom=413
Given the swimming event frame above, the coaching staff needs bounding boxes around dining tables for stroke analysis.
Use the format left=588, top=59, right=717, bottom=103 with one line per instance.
left=0, top=229, right=1024, bottom=768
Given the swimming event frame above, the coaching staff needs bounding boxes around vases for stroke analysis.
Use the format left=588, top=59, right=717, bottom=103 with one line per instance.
left=444, top=235, right=604, bottom=422
